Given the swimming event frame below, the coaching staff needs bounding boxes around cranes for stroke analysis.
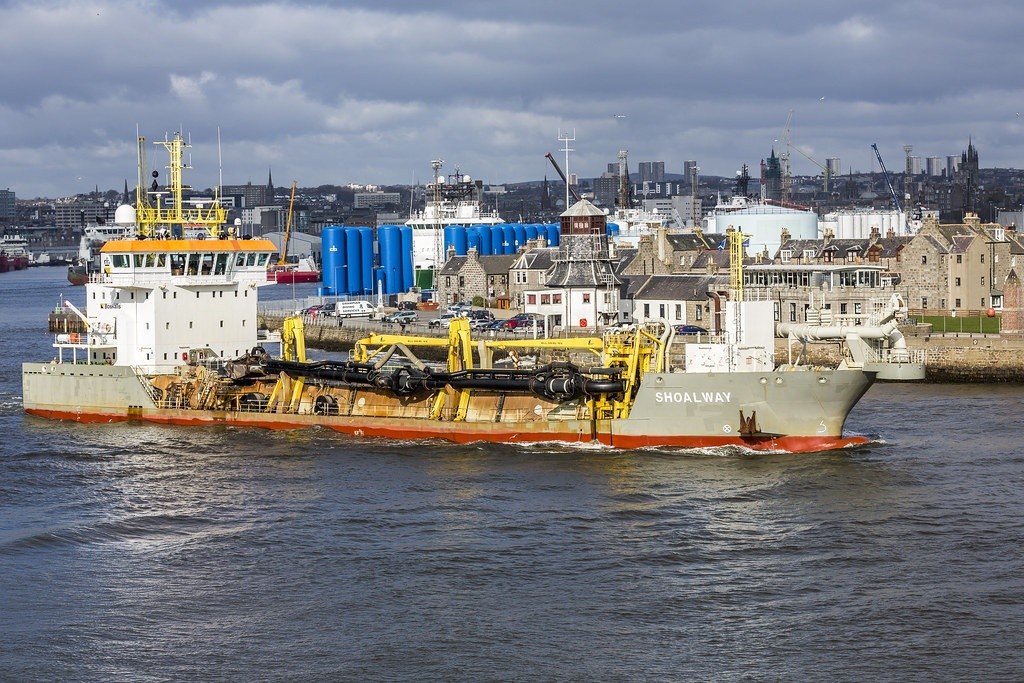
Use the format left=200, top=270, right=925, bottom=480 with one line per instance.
left=787, top=143, right=902, bottom=212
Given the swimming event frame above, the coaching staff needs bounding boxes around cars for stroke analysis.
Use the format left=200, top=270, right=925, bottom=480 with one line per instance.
left=398, top=301, right=417, bottom=311
left=429, top=301, right=548, bottom=330
left=382, top=311, right=419, bottom=324
left=298, top=300, right=336, bottom=319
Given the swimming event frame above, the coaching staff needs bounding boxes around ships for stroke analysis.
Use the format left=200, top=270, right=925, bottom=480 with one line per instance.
left=22, top=120, right=880, bottom=452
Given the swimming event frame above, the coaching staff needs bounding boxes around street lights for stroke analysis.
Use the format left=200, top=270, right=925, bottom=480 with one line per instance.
left=438, top=176, right=445, bottom=201
left=104, top=202, right=109, bottom=222
left=736, top=171, right=742, bottom=182
left=463, top=175, right=471, bottom=190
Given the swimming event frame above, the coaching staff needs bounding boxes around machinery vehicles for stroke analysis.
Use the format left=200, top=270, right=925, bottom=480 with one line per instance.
left=277, top=178, right=297, bottom=265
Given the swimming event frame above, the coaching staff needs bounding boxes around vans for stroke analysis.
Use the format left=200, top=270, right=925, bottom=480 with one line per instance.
left=335, top=301, right=377, bottom=317
